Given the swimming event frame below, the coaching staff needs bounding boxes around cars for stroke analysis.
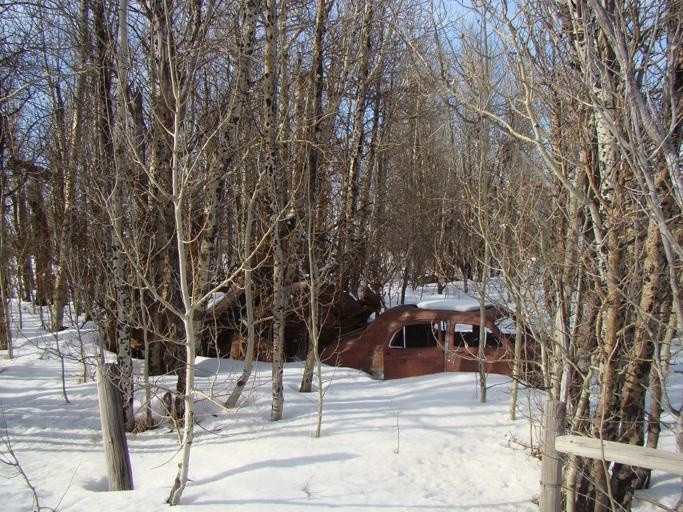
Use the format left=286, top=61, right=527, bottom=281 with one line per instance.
left=111, top=242, right=383, bottom=361
left=318, top=298, right=549, bottom=389
